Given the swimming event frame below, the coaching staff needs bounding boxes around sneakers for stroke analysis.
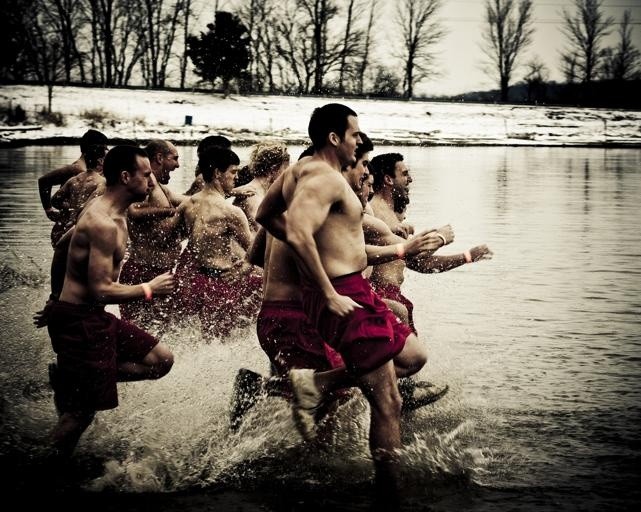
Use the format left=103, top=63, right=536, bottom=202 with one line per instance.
left=46, top=360, right=57, bottom=389
left=50, top=450, right=106, bottom=478
left=229, top=368, right=265, bottom=434
left=401, top=377, right=453, bottom=413
left=289, top=364, right=327, bottom=445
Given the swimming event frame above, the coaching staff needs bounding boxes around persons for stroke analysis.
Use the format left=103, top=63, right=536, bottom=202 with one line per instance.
left=229, top=104, right=494, bottom=503
left=32, top=128, right=292, bottom=469
